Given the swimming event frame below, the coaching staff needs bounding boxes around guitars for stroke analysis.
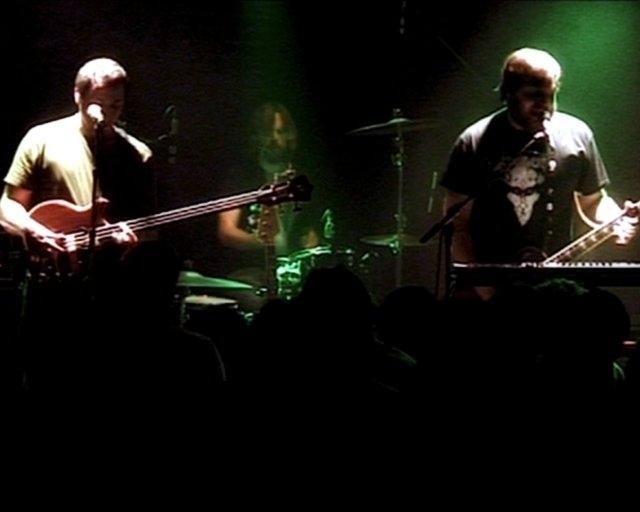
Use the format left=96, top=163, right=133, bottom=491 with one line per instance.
left=517, top=202, right=640, bottom=261
left=249, top=185, right=279, bottom=299
left=25, top=176, right=315, bottom=275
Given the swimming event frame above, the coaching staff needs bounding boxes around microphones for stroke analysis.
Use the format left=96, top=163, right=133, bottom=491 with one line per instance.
left=87, top=104, right=109, bottom=126
left=517, top=129, right=547, bottom=162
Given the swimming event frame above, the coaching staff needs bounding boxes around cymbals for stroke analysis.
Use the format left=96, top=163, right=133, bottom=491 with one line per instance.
left=177, top=271, right=253, bottom=294
left=353, top=118, right=442, bottom=136
left=359, top=231, right=430, bottom=246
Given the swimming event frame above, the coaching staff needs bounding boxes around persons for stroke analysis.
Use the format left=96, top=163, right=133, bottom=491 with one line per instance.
left=437, top=46, right=640, bottom=296
left=1, top=54, right=161, bottom=268
left=0, top=235, right=640, bottom=511
left=214, top=100, right=328, bottom=313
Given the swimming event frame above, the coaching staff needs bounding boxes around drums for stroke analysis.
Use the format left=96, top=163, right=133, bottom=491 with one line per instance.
left=288, top=247, right=375, bottom=298
left=184, top=296, right=237, bottom=322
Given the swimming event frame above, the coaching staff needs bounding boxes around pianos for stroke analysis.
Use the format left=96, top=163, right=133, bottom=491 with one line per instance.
left=451, top=261, right=640, bottom=288
